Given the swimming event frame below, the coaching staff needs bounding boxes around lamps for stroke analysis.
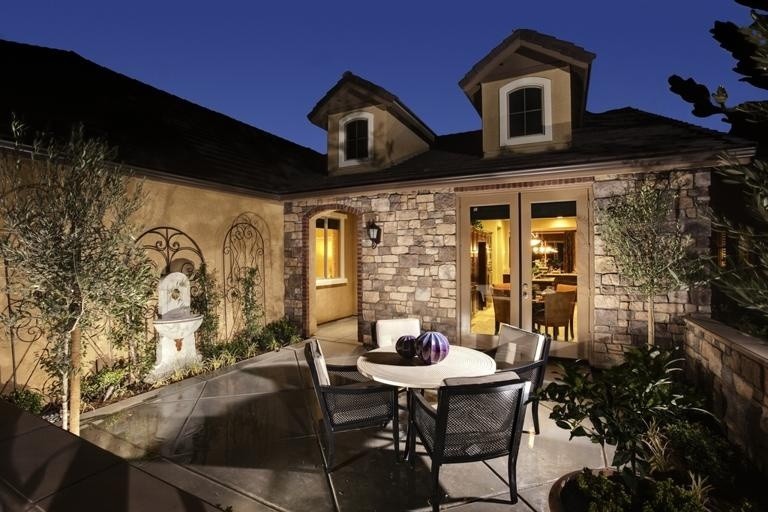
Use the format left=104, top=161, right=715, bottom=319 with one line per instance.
left=366, top=220, right=379, bottom=250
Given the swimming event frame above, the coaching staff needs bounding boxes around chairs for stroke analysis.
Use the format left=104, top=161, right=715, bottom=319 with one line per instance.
left=490, top=283, right=577, bottom=341
left=303, top=317, right=550, bottom=512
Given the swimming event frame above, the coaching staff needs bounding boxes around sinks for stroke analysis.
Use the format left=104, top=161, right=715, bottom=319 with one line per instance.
left=153, top=312, right=204, bottom=340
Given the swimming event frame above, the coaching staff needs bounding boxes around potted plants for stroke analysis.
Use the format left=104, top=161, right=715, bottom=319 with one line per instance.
left=522, top=339, right=758, bottom=512
